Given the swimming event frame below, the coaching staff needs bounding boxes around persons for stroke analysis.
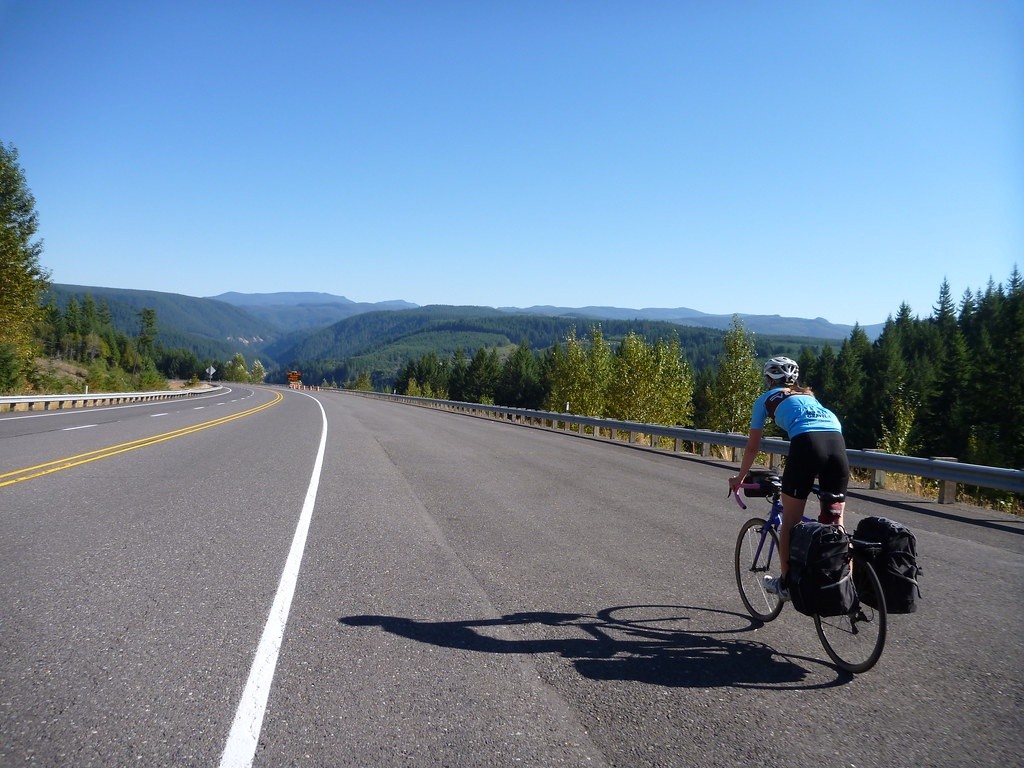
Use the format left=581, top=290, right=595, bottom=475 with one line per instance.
left=728, top=356, right=850, bottom=601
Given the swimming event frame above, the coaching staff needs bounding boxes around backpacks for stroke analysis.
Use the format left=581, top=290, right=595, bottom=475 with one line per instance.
left=785, top=521, right=859, bottom=618
left=852, top=516, right=918, bottom=614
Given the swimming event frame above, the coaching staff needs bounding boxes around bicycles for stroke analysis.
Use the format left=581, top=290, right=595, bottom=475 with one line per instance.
left=727, top=467, right=889, bottom=674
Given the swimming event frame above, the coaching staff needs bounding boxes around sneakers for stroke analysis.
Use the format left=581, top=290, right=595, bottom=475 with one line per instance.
left=763, top=575, right=791, bottom=602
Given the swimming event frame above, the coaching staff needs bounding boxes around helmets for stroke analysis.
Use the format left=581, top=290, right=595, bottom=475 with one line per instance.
left=763, top=357, right=799, bottom=380
